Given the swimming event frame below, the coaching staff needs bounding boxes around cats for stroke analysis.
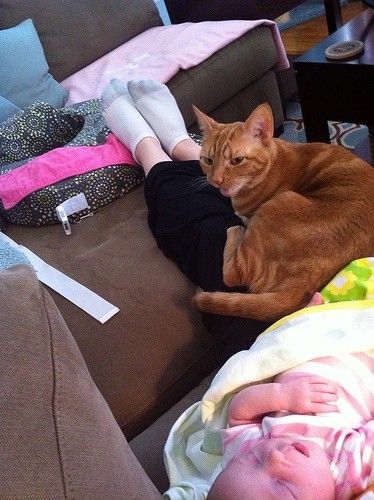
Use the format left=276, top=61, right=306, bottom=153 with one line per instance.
left=191, top=102, right=374, bottom=322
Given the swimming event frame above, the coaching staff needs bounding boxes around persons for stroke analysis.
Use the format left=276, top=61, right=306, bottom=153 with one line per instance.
left=100, top=78, right=325, bottom=357
left=162, top=256, right=374, bottom=500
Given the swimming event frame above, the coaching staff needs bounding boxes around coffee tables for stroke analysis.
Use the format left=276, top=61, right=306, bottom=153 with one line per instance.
left=292, top=7, right=374, bottom=144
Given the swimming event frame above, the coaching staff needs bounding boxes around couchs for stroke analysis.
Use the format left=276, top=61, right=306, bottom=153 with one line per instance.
left=0, top=0, right=287, bottom=500
